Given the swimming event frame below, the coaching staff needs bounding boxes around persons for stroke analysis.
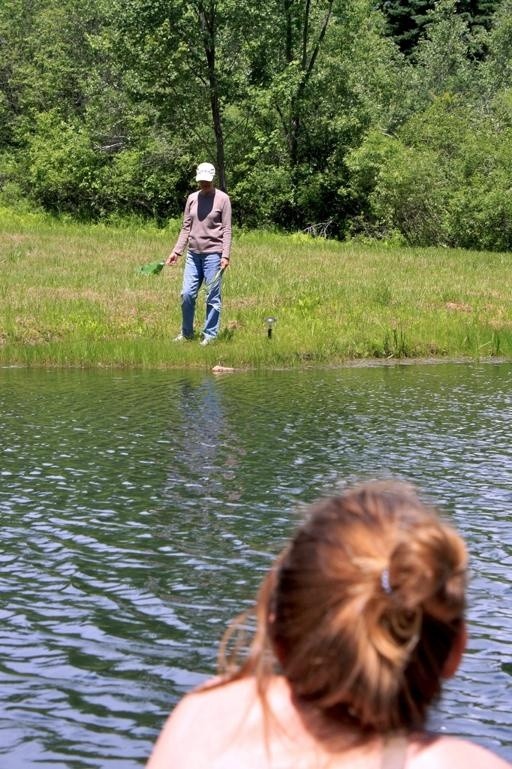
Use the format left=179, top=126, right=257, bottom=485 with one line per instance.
left=153, top=374, right=242, bottom=571
left=167, top=162, right=233, bottom=346
left=146, top=482, right=510, bottom=769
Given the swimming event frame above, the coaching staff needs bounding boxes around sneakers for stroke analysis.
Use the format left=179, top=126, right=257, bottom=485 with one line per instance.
left=199, top=337, right=209, bottom=345
left=173, top=330, right=192, bottom=341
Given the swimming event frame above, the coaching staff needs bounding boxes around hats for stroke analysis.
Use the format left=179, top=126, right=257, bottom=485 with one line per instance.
left=194, top=162, right=216, bottom=181
